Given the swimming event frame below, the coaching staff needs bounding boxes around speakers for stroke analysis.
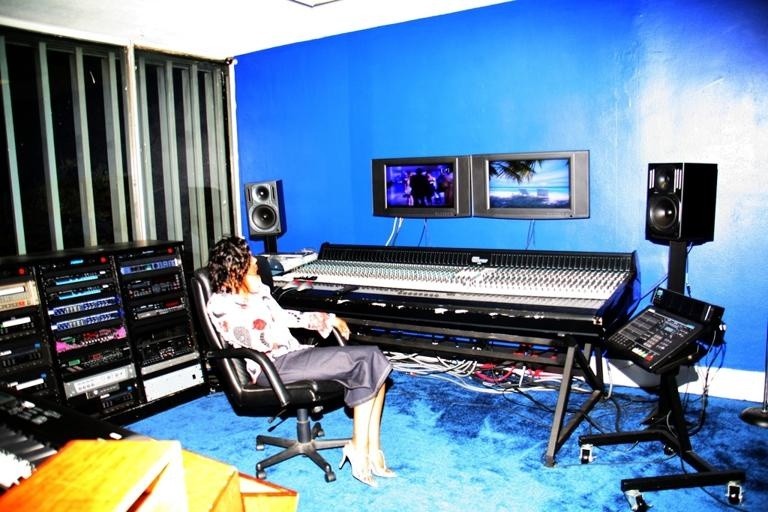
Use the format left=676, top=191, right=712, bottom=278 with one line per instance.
left=244, top=180, right=287, bottom=241
left=645, top=162, right=717, bottom=242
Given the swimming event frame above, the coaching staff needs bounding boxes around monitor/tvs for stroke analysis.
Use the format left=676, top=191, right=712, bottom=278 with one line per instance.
left=471, top=150, right=589, bottom=221
left=372, top=155, right=470, bottom=219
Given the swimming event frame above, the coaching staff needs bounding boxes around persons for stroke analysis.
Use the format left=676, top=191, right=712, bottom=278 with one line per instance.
left=403, top=164, right=453, bottom=208
left=205, top=233, right=396, bottom=489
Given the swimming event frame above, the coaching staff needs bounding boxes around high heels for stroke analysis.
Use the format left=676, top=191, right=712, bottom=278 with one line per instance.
left=369, top=449, right=396, bottom=478
left=339, top=441, right=376, bottom=487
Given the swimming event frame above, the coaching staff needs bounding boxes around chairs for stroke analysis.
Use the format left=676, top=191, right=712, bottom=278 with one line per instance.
left=188, top=257, right=366, bottom=483
